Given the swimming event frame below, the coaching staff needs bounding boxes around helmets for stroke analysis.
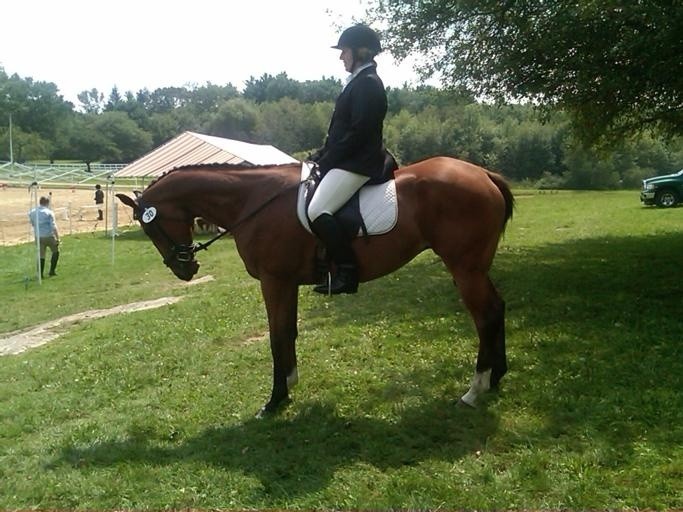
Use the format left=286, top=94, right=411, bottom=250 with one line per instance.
left=330, top=25, right=382, bottom=52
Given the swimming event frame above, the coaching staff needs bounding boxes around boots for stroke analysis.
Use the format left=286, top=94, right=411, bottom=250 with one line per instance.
left=41, top=258, right=45, bottom=280
left=310, top=213, right=358, bottom=295
left=48, top=260, right=57, bottom=275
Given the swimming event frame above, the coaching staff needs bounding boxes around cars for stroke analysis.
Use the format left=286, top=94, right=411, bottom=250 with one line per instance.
left=640, top=168, right=683, bottom=208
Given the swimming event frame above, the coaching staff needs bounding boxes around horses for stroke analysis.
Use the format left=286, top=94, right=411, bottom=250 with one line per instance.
left=114, top=156, right=517, bottom=421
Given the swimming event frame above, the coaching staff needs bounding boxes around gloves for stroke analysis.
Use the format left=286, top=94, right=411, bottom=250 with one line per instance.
left=56, top=239, right=62, bottom=247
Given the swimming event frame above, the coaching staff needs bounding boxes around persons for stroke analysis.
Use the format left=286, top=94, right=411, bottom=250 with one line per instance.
left=196, top=218, right=211, bottom=235
left=28, top=197, right=60, bottom=279
left=93, top=184, right=104, bottom=221
left=300, top=22, right=389, bottom=295
